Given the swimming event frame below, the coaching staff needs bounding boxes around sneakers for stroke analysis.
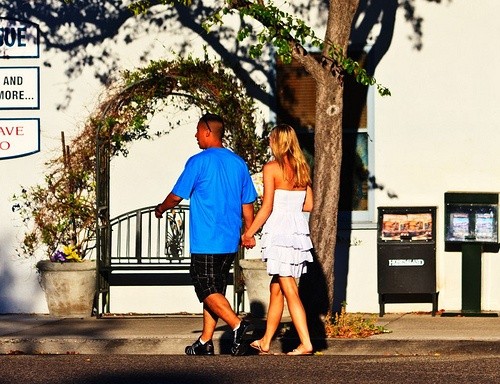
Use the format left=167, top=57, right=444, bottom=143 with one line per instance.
left=185, top=336, right=214, bottom=356
left=230, top=321, right=254, bottom=356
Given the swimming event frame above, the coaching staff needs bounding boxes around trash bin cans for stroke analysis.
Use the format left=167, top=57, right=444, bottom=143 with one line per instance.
left=377, top=206, right=435, bottom=294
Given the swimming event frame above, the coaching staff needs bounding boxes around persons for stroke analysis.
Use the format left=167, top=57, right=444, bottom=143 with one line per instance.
left=241, top=124, right=314, bottom=356
left=154, top=113, right=258, bottom=357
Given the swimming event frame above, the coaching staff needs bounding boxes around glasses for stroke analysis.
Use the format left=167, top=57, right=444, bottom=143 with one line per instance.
left=202, top=113, right=212, bottom=132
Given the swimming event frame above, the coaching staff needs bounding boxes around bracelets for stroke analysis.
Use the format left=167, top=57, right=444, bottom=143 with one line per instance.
left=156, top=204, right=165, bottom=214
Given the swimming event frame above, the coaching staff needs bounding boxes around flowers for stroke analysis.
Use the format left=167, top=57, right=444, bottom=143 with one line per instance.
left=10, top=164, right=110, bottom=288
left=247, top=173, right=263, bottom=240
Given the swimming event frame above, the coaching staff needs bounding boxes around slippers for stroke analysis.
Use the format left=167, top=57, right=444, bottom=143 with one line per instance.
left=250, top=340, right=269, bottom=353
left=287, top=349, right=313, bottom=356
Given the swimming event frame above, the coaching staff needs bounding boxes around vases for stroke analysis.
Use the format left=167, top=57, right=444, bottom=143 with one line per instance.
left=38, top=260, right=99, bottom=320
left=239, top=260, right=291, bottom=319
